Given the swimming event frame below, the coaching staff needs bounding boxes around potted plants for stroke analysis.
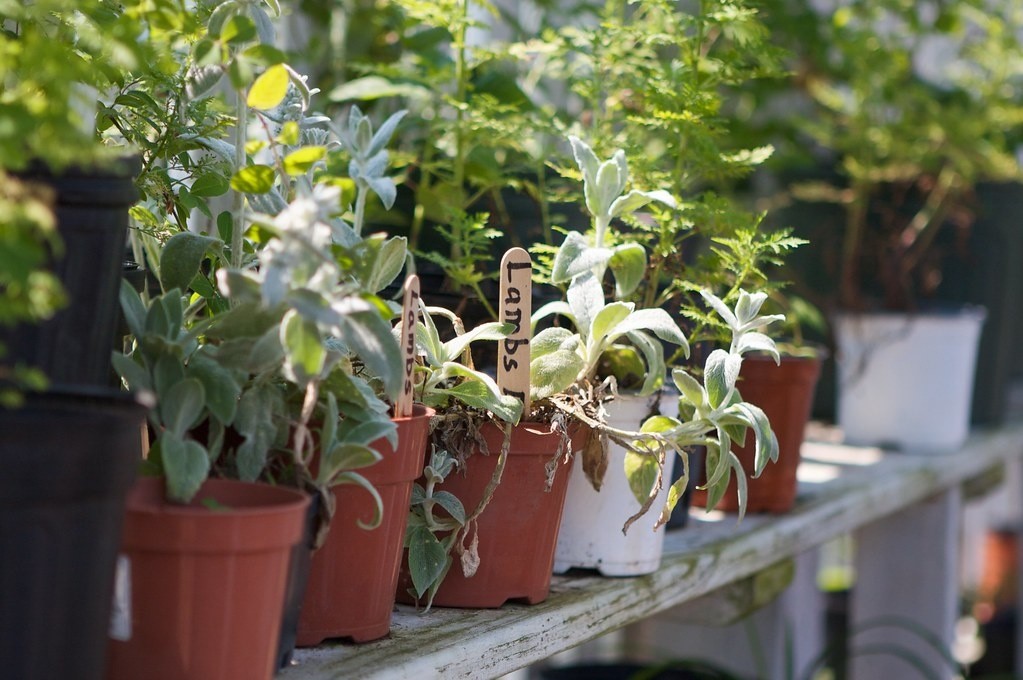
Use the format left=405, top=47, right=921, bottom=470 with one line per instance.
left=0, top=0, right=1023, bottom=678
